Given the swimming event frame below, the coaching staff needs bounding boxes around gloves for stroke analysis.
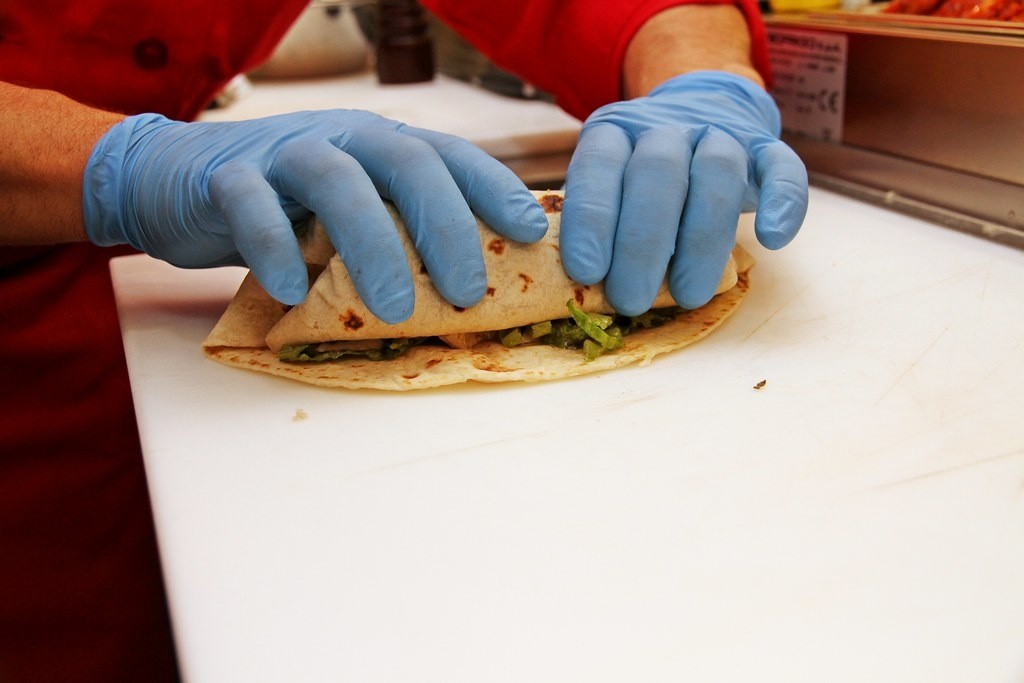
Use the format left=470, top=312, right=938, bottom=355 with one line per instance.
left=559, top=71, right=809, bottom=316
left=83, top=109, right=548, bottom=324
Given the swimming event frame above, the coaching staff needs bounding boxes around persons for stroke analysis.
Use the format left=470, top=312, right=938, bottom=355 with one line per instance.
left=0, top=0, right=809, bottom=683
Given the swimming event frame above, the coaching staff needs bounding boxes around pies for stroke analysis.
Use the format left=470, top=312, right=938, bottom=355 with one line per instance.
left=199, top=191, right=756, bottom=391
